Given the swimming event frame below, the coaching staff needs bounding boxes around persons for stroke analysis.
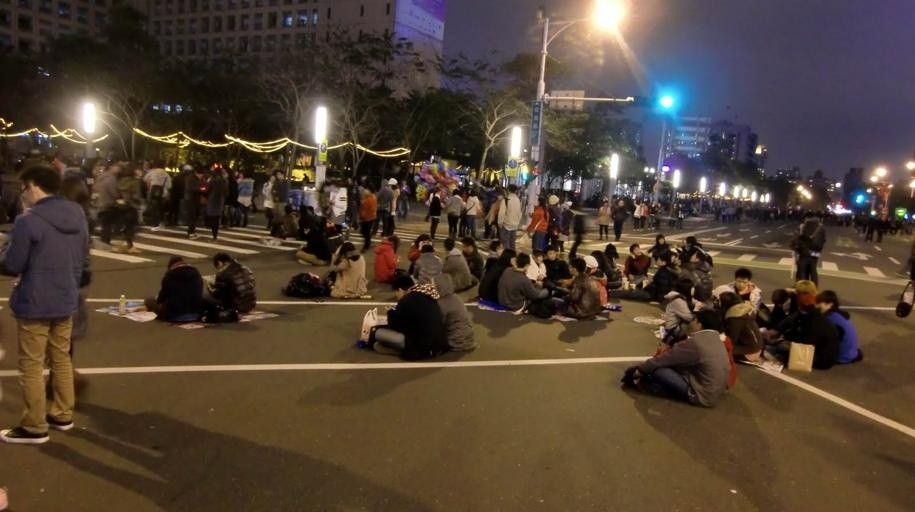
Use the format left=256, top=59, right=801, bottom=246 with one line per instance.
left=0, top=165, right=91, bottom=445
left=0, top=147, right=914, bottom=409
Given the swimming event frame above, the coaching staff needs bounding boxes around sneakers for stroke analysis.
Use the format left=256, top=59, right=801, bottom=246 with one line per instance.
left=188, top=233, right=200, bottom=241
left=152, top=223, right=161, bottom=232
left=45, top=414, right=74, bottom=430
left=128, top=247, right=142, bottom=253
left=0, top=425, right=50, bottom=444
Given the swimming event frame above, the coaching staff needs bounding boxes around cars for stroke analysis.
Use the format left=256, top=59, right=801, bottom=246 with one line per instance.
left=744, top=206, right=781, bottom=222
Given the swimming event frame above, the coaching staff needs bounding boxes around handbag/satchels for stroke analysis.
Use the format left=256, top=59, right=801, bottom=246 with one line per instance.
left=702, top=253, right=714, bottom=271
left=445, top=205, right=457, bottom=214
left=786, top=341, right=816, bottom=373
left=151, top=185, right=163, bottom=200
left=528, top=228, right=537, bottom=238
left=895, top=287, right=915, bottom=318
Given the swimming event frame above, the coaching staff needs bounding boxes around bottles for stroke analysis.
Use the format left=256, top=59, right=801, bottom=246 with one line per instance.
left=660, top=326, right=666, bottom=340
left=118, top=295, right=126, bottom=315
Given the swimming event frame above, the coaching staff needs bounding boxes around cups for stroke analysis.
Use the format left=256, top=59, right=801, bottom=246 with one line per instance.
left=622, top=278, right=627, bottom=288
left=624, top=282, right=629, bottom=290
left=643, top=280, right=648, bottom=288
left=630, top=283, right=635, bottom=288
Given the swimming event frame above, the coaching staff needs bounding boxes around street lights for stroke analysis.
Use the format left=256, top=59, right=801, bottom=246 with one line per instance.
left=641, top=165, right=772, bottom=216
left=855, top=160, right=915, bottom=219
left=312, top=105, right=329, bottom=192
left=507, top=125, right=522, bottom=187
left=80, top=99, right=100, bottom=162
left=784, top=183, right=813, bottom=222
left=525, top=0, right=635, bottom=216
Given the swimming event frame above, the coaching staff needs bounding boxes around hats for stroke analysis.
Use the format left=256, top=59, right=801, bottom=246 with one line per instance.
left=549, top=195, right=559, bottom=206
left=561, top=201, right=573, bottom=209
left=387, top=178, right=398, bottom=185
